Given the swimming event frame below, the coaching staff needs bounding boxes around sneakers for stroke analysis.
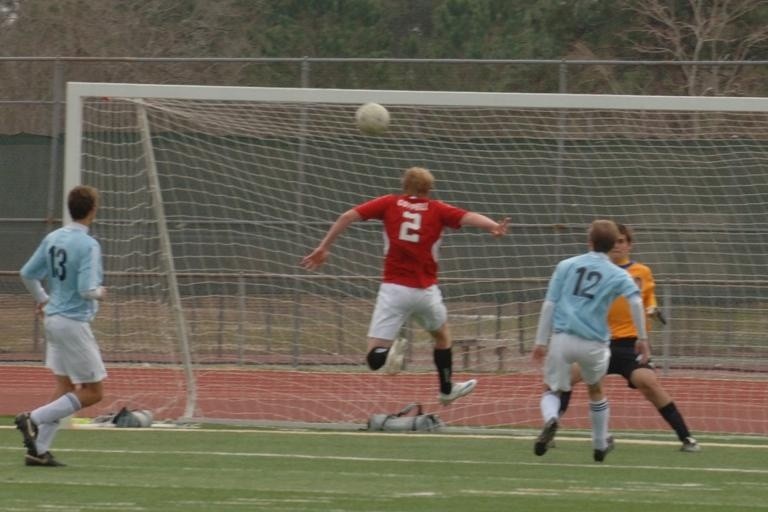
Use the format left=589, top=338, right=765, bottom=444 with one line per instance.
left=438, top=379, right=477, bottom=404
left=681, top=436, right=699, bottom=451
left=594, top=436, right=614, bottom=462
left=25, top=451, right=60, bottom=466
left=384, top=337, right=408, bottom=375
left=14, top=412, right=37, bottom=450
left=534, top=417, right=557, bottom=455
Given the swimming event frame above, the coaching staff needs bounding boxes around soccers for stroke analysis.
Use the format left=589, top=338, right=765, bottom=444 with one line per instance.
left=356, top=104, right=389, bottom=135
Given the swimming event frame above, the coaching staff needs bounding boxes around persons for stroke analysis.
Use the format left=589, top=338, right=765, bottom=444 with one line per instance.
left=529, top=215, right=652, bottom=463
left=298, top=162, right=514, bottom=407
left=10, top=184, right=109, bottom=467
left=555, top=222, right=703, bottom=455
